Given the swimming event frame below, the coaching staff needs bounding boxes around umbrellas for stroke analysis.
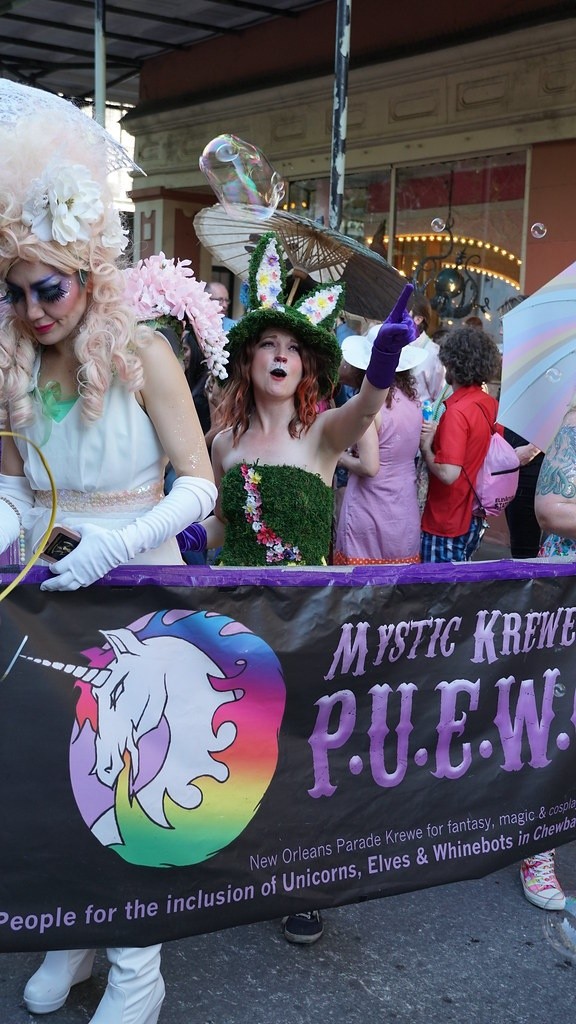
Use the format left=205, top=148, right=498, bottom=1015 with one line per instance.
left=193, top=203, right=409, bottom=320
left=494, top=260, right=576, bottom=454
left=0, top=78, right=149, bottom=179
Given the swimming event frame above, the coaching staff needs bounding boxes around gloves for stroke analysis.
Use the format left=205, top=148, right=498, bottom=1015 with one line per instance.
left=39, top=476, right=219, bottom=593
left=366, top=284, right=417, bottom=389
left=176, top=523, right=207, bottom=553
left=0, top=473, right=34, bottom=554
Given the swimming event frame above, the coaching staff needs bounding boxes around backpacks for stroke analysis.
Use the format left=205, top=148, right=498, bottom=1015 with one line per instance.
left=461, top=400, right=520, bottom=519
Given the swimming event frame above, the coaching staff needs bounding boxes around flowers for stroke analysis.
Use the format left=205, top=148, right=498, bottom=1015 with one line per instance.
left=240, top=463, right=306, bottom=568
left=116, top=251, right=231, bottom=384
left=19, top=164, right=103, bottom=244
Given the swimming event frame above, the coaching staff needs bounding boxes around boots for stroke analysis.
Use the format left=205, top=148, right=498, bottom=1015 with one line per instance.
left=23, top=947, right=95, bottom=1014
left=87, top=943, right=165, bottom=1024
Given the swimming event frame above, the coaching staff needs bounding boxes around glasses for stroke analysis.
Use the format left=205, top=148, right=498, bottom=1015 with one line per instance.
left=211, top=297, right=231, bottom=306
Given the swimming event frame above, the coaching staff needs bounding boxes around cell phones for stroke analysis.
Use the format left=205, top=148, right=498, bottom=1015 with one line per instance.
left=32, top=523, right=82, bottom=564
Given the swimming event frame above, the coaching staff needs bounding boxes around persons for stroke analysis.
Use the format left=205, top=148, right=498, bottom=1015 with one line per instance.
left=176, top=285, right=416, bottom=944
left=205, top=282, right=238, bottom=330
left=181, top=323, right=212, bottom=434
left=496, top=384, right=545, bottom=558
left=522, top=390, right=576, bottom=910
left=405, top=292, right=447, bottom=520
left=330, top=322, right=423, bottom=566
left=419, top=324, right=504, bottom=562
left=0, top=104, right=218, bottom=1024
left=328, top=314, right=363, bottom=525
left=417, top=380, right=488, bottom=519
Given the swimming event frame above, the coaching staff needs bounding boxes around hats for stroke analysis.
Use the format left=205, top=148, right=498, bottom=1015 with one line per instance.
left=215, top=232, right=347, bottom=404
left=341, top=323, right=428, bottom=372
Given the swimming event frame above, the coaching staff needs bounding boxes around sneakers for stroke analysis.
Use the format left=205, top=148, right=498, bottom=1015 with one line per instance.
left=520, top=848, right=566, bottom=910
left=281, top=909, right=324, bottom=943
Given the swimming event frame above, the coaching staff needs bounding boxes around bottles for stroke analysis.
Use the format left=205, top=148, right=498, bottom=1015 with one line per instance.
left=422, top=400, right=433, bottom=423
